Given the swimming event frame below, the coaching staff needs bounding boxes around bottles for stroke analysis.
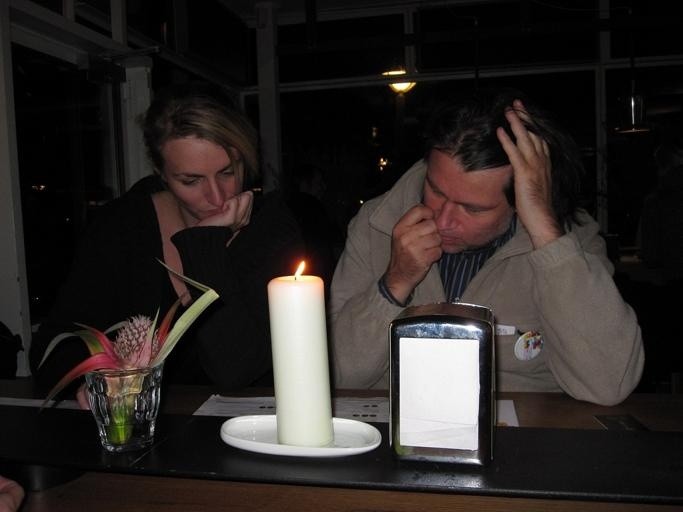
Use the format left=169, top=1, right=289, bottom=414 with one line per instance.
left=619, top=80, right=646, bottom=127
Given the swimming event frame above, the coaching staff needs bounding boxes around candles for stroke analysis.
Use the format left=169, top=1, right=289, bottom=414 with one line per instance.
left=266, top=258, right=336, bottom=448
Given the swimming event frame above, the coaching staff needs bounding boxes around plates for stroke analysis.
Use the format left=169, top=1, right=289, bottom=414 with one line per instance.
left=220, top=415, right=382, bottom=458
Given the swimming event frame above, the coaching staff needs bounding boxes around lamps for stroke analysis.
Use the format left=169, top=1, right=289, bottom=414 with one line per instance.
left=613, top=0, right=649, bottom=134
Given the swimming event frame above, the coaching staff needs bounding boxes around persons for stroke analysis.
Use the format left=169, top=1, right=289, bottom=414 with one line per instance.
left=0, top=475, right=25, bottom=512
left=29, top=82, right=320, bottom=411
left=330, top=93, right=646, bottom=407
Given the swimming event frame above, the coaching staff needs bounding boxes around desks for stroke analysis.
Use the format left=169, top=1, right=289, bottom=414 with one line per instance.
left=1, top=376, right=683, bottom=512
